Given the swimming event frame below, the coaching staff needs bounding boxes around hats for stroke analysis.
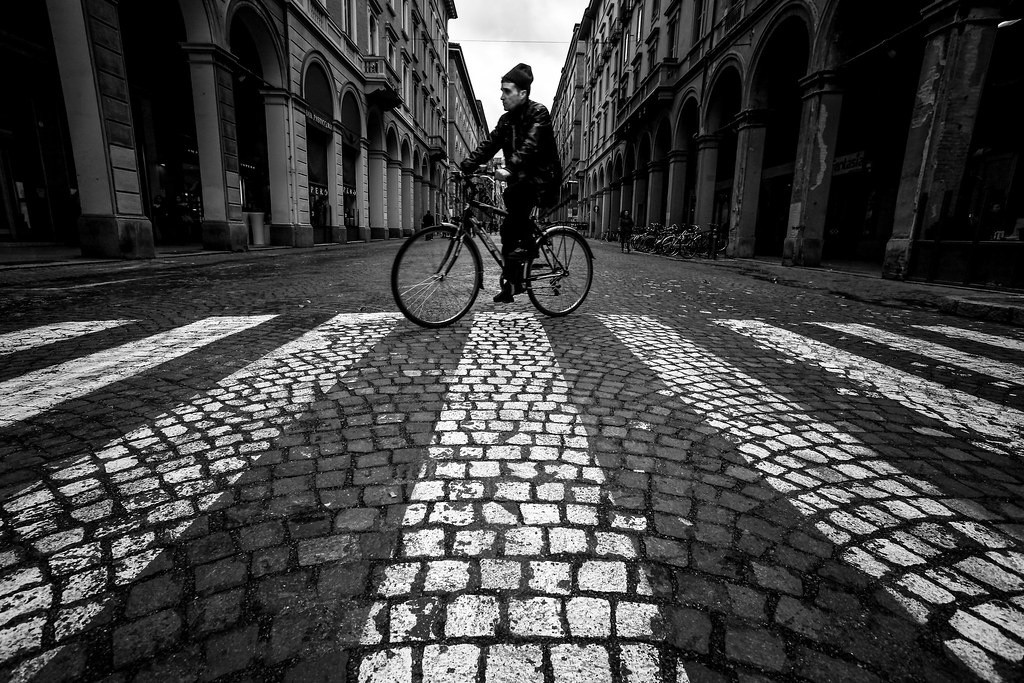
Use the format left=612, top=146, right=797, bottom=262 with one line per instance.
left=503, top=63, right=534, bottom=96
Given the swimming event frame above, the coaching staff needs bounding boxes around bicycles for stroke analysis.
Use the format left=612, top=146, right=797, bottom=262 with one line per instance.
left=390, top=164, right=598, bottom=329
left=602, top=221, right=731, bottom=260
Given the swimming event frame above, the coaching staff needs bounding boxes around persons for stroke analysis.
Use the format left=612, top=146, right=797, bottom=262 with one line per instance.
left=423, top=210, right=435, bottom=241
left=620, top=210, right=634, bottom=253
left=460, top=63, right=563, bottom=302
left=441, top=215, right=448, bottom=238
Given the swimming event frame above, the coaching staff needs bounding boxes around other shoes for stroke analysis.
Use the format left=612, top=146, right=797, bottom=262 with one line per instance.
left=509, top=247, right=539, bottom=260
left=627, top=249, right=631, bottom=253
left=621, top=250, right=624, bottom=253
left=493, top=292, right=514, bottom=304
